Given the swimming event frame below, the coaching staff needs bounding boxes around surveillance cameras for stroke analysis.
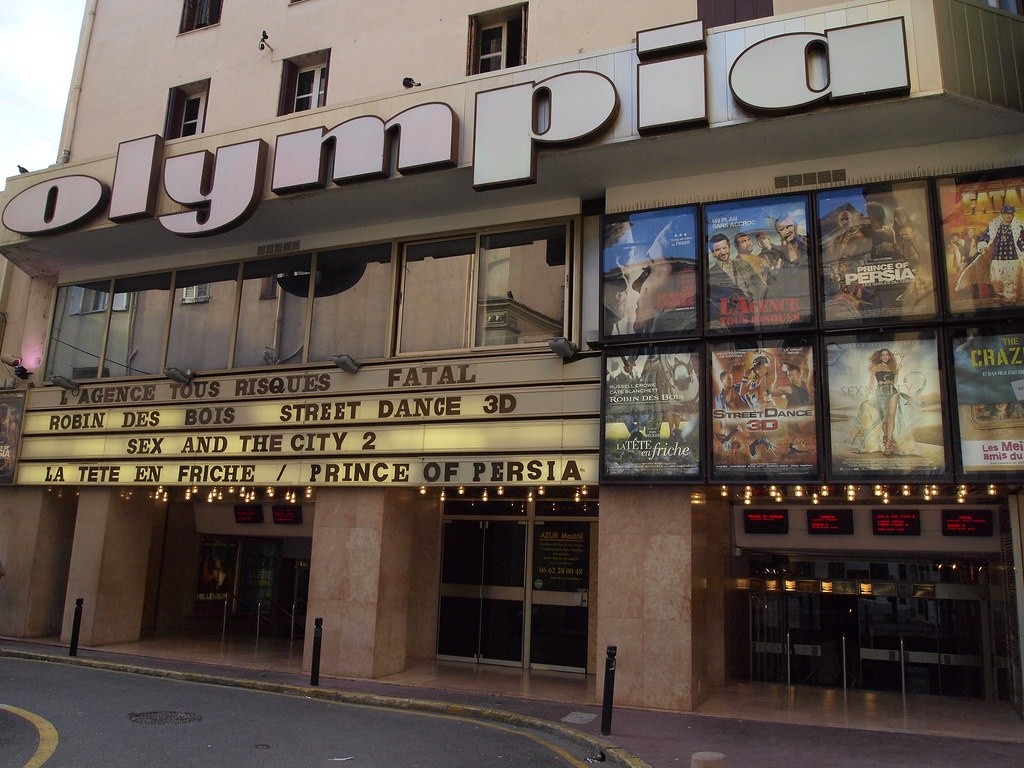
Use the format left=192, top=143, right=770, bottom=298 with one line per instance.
left=1, top=354, right=20, bottom=367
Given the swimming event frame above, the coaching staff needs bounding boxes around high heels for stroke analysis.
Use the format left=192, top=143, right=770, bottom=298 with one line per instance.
left=883, top=436, right=894, bottom=456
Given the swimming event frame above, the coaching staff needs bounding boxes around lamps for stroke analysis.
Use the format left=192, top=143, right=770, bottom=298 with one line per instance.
left=331, top=353, right=362, bottom=374
left=546, top=337, right=578, bottom=358
left=164, top=367, right=193, bottom=384
left=48, top=374, right=80, bottom=391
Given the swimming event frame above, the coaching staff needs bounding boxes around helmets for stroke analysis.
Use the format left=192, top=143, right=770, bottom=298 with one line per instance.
left=859, top=202, right=885, bottom=224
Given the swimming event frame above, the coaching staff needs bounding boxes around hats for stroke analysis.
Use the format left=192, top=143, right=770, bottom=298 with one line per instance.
left=749, top=356, right=769, bottom=370
left=1001, top=205, right=1015, bottom=214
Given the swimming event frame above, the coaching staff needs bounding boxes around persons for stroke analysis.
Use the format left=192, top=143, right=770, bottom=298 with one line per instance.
left=832, top=206, right=930, bottom=315
left=603, top=212, right=697, bottom=335
left=712, top=213, right=810, bottom=327
left=949, top=204, right=1024, bottom=303
left=715, top=348, right=811, bottom=462
left=865, top=348, right=902, bottom=455
